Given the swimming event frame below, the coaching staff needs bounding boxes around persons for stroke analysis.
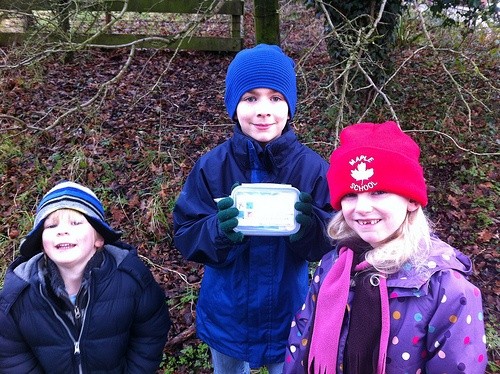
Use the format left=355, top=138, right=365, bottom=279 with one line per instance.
left=0, top=180, right=173, bottom=374
left=282, top=120, right=487, bottom=374
left=171, top=43, right=346, bottom=373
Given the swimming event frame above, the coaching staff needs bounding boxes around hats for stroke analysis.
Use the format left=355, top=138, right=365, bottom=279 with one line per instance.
left=326, top=121, right=428, bottom=207
left=224, top=44, right=297, bottom=121
left=19, top=181, right=123, bottom=258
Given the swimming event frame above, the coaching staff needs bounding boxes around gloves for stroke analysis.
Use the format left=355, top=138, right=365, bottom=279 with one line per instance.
left=217, top=197, right=243, bottom=244
left=290, top=192, right=315, bottom=245
